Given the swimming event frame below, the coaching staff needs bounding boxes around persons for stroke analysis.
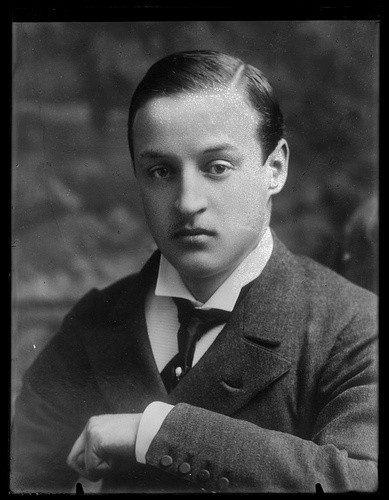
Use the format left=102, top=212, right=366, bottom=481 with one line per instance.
left=9, top=48, right=378, bottom=493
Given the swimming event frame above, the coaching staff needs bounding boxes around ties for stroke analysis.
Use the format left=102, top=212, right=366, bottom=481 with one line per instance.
left=159, top=296, right=231, bottom=394
